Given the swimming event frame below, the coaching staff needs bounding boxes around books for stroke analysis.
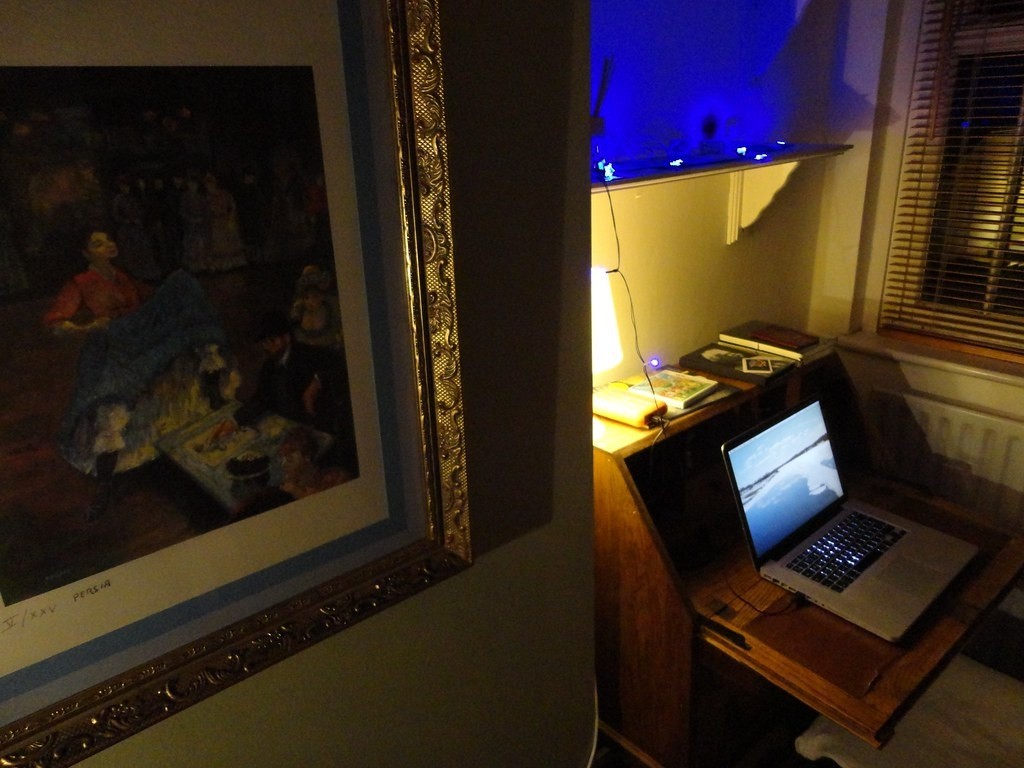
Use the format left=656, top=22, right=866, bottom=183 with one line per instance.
left=626, top=319, right=835, bottom=410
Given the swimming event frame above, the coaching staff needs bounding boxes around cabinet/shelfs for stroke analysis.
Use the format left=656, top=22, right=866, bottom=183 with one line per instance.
left=590, top=321, right=1024, bottom=768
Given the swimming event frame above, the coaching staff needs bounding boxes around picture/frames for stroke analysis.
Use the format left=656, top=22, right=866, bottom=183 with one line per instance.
left=0, top=0, right=476, bottom=768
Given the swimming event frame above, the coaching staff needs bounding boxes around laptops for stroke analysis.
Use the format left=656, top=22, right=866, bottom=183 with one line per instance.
left=721, top=389, right=979, bottom=641
